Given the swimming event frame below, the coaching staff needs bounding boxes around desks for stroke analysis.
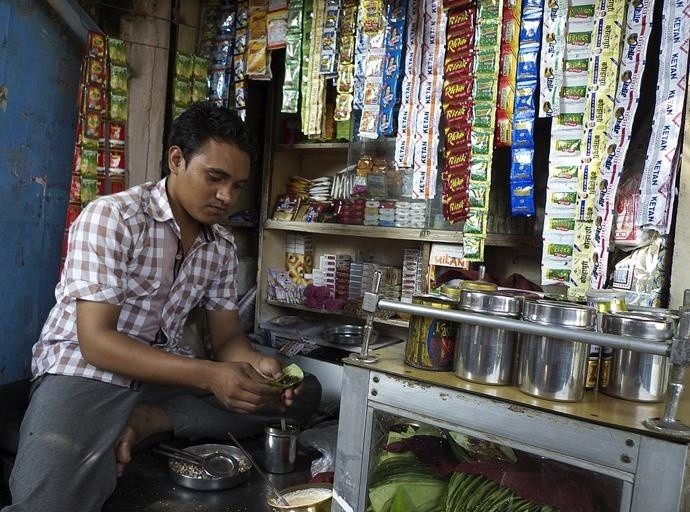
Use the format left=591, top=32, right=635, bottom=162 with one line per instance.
left=331, top=340, right=690, bottom=512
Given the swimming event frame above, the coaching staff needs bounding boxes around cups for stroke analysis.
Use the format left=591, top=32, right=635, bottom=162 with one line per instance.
left=265, top=422, right=301, bottom=474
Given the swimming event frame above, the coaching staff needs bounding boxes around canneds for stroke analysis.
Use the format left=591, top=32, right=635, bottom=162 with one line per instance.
left=404, top=280, right=499, bottom=372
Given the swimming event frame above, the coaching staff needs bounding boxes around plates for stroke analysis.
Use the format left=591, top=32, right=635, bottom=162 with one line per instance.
left=324, top=324, right=381, bottom=347
left=166, top=443, right=254, bottom=491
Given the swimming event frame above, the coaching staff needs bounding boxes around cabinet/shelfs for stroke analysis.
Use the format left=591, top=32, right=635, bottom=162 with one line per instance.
left=254, top=73, right=541, bottom=337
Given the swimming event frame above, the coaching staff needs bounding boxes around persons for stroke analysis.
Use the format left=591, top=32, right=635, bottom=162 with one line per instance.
left=1, top=99, right=322, bottom=511
